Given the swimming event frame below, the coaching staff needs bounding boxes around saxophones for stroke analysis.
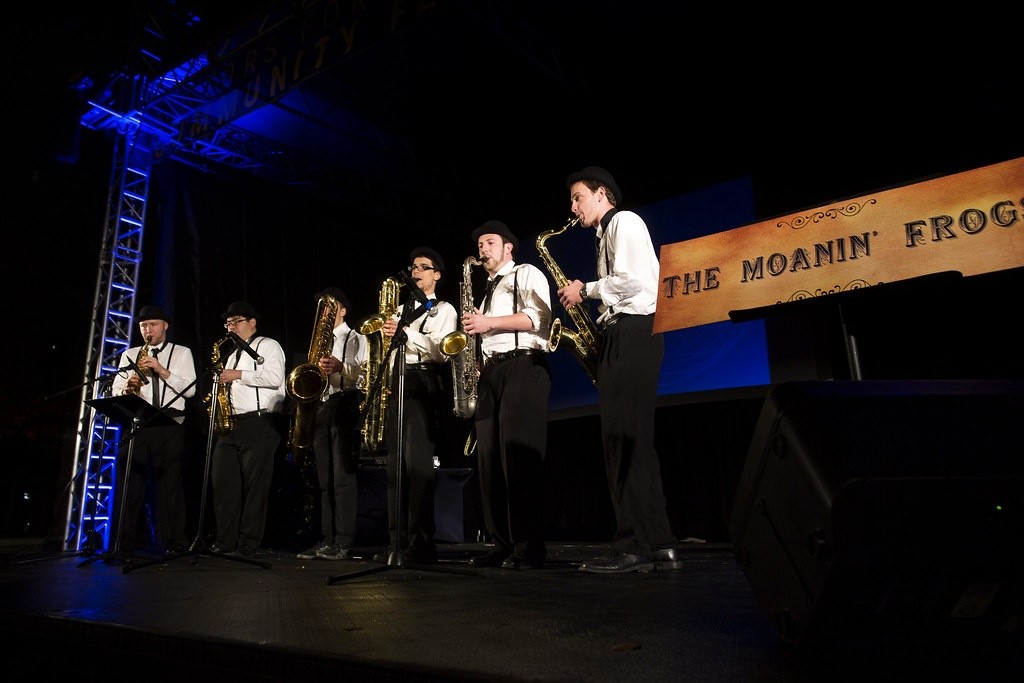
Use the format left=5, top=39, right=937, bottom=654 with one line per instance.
left=439, top=254, right=492, bottom=420
left=536, top=215, right=601, bottom=387
left=285, top=295, right=338, bottom=462
left=359, top=269, right=420, bottom=454
left=200, top=333, right=239, bottom=436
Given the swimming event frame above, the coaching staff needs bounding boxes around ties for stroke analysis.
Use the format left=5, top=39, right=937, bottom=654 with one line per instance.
left=322, top=334, right=335, bottom=402
left=408, top=298, right=438, bottom=328
left=151, top=339, right=168, bottom=407
left=227, top=332, right=259, bottom=416
left=476, top=262, right=517, bottom=371
left=595, top=207, right=620, bottom=261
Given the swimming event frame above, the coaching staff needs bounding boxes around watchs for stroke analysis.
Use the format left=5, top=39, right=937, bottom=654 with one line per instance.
left=580, top=283, right=592, bottom=303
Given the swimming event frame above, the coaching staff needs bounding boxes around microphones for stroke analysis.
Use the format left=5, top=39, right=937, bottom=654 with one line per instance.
left=128, top=358, right=149, bottom=384
left=230, top=332, right=259, bottom=358
left=399, top=271, right=432, bottom=308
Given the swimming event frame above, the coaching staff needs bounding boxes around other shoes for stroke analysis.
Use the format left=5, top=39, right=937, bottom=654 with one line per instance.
left=399, top=551, right=437, bottom=564
left=196, top=544, right=235, bottom=559
left=296, top=545, right=329, bottom=559
left=237, top=544, right=256, bottom=558
left=318, top=544, right=352, bottom=560
left=371, top=551, right=396, bottom=563
left=161, top=542, right=185, bottom=555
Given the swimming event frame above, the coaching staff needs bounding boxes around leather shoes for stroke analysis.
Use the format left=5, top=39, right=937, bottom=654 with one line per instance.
left=653, top=547, right=683, bottom=571
left=578, top=548, right=655, bottom=573
left=468, top=552, right=508, bottom=567
left=502, top=553, right=545, bottom=569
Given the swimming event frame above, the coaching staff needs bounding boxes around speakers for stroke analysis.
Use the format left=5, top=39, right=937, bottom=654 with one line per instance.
left=728, top=379, right=1024, bottom=644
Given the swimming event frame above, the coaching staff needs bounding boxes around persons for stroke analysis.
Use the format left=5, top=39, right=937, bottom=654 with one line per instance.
left=104, top=304, right=197, bottom=562
left=372, top=242, right=458, bottom=566
left=461, top=220, right=553, bottom=570
left=296, top=284, right=370, bottom=559
left=557, top=165, right=683, bottom=575
left=198, top=298, right=287, bottom=559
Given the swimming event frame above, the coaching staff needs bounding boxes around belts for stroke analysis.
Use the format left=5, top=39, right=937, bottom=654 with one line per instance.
left=597, top=313, right=627, bottom=334
left=406, top=364, right=434, bottom=370
left=485, top=348, right=538, bottom=367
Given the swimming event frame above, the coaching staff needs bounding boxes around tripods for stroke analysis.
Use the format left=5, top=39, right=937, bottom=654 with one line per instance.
left=15, top=382, right=164, bottom=563
left=120, top=345, right=273, bottom=573
left=79, top=392, right=186, bottom=567
left=323, top=297, right=487, bottom=584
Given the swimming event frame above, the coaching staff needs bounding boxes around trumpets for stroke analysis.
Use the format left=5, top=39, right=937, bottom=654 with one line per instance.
left=122, top=335, right=152, bottom=397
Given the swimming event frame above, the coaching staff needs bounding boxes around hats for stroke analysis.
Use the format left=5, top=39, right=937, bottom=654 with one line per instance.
left=410, top=247, right=445, bottom=271
left=220, top=301, right=257, bottom=321
left=134, top=306, right=172, bottom=324
left=566, top=166, right=622, bottom=207
left=471, top=220, right=519, bottom=248
